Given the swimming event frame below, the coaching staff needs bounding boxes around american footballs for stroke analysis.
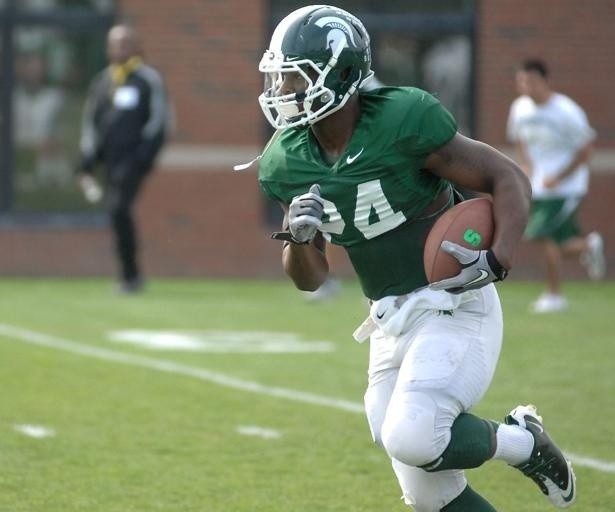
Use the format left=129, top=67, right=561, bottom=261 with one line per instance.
left=422, top=197, right=493, bottom=283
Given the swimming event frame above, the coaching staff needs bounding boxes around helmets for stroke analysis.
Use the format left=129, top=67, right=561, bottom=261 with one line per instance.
left=256, top=4, right=375, bottom=130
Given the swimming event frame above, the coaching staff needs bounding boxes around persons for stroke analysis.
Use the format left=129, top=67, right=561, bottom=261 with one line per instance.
left=499, top=54, right=608, bottom=316
left=248, top=3, right=581, bottom=511
left=8, top=48, right=70, bottom=180
left=73, top=24, right=169, bottom=293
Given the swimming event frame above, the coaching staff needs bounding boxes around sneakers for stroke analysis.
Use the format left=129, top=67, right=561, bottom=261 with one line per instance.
left=502, top=403, right=577, bottom=508
left=531, top=295, right=567, bottom=317
left=582, top=232, right=608, bottom=283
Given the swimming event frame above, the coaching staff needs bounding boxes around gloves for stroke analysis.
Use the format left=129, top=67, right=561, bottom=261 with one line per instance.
left=429, top=240, right=507, bottom=295
left=287, top=183, right=327, bottom=245
